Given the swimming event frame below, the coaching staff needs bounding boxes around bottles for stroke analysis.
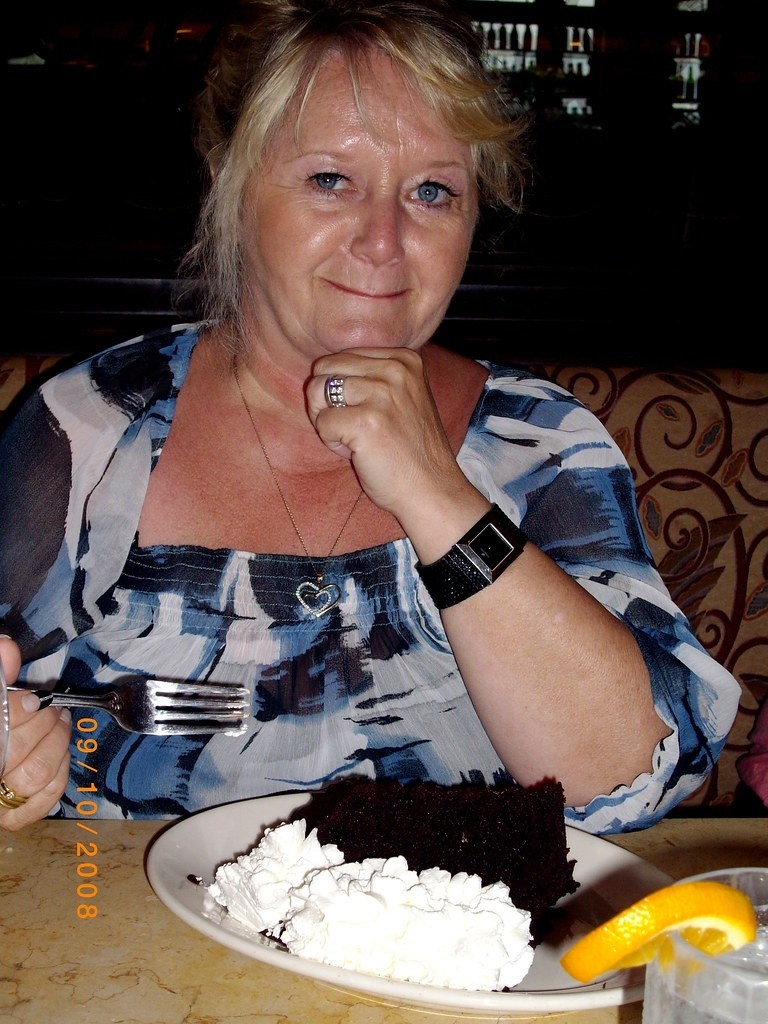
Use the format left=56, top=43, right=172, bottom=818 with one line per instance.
left=668, top=33, right=705, bottom=126
left=477, top=22, right=536, bottom=74
left=564, top=29, right=593, bottom=118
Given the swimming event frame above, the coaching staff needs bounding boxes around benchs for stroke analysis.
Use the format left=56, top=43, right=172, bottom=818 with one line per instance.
left=0, top=352, right=768, bottom=817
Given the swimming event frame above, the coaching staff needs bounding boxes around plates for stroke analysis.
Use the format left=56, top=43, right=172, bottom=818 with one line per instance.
left=145, top=791, right=675, bottom=1017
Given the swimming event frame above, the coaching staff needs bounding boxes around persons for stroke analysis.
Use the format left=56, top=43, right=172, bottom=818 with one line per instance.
left=1, top=2, right=737, bottom=835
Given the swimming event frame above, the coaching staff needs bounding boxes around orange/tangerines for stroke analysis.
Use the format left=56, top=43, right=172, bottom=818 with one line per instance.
left=559, top=882, right=759, bottom=984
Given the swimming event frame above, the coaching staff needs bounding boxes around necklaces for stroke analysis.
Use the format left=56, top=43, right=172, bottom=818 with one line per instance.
left=228, top=349, right=362, bottom=615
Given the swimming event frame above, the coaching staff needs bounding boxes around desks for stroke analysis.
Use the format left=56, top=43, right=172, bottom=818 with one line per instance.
left=1, top=817, right=768, bottom=1024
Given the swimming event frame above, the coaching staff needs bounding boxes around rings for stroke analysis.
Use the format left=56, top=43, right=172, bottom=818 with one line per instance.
left=0, top=779, right=28, bottom=810
left=324, top=371, right=350, bottom=408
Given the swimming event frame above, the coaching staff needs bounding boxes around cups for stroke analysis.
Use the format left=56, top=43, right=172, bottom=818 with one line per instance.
left=0, top=658, right=10, bottom=772
left=644, top=867, right=768, bottom=1024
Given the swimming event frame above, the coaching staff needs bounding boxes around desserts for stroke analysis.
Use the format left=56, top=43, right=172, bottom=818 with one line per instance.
left=202, top=763, right=581, bottom=993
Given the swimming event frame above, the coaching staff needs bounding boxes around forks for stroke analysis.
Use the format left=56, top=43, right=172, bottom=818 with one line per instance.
left=7, top=679, right=250, bottom=736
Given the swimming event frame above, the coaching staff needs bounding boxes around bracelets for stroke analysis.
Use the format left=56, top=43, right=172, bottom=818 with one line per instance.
left=412, top=503, right=529, bottom=611
